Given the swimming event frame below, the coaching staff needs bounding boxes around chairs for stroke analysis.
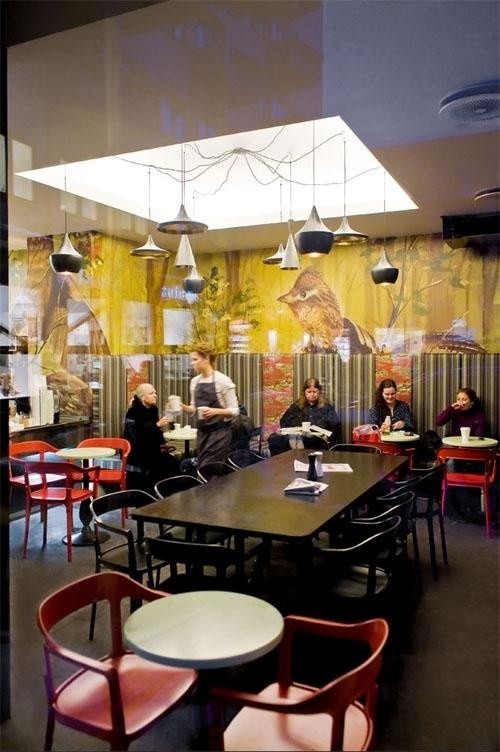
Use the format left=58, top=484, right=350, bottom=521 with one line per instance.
left=8, top=439, right=63, bottom=524
left=437, top=448, right=498, bottom=543
left=87, top=449, right=274, bottom=646
left=351, top=423, right=414, bottom=483
left=208, top=612, right=390, bottom=751
left=75, top=437, right=131, bottom=531
left=37, top=569, right=198, bottom=752
left=22, top=459, right=102, bottom=563
left=307, top=462, right=449, bottom=622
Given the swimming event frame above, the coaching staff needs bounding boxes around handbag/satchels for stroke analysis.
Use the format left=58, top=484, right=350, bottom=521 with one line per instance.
left=352, top=423, right=382, bottom=443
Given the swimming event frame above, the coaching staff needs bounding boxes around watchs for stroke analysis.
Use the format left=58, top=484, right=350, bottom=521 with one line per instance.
left=156, top=422, right=161, bottom=427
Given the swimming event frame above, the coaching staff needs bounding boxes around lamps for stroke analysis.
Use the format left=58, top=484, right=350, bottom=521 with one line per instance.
left=292, top=120, right=336, bottom=259
left=329, top=134, right=369, bottom=247
left=438, top=79, right=498, bottom=126
left=156, top=145, right=208, bottom=236
left=171, top=234, right=197, bottom=268
left=261, top=178, right=285, bottom=266
left=48, top=165, right=84, bottom=276
left=371, top=168, right=401, bottom=285
left=474, top=185, right=498, bottom=205
left=181, top=234, right=206, bottom=295
left=129, top=169, right=171, bottom=261
left=280, top=160, right=301, bottom=272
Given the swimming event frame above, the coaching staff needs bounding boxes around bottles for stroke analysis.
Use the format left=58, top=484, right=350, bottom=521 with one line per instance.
left=384, top=416, right=391, bottom=435
left=305, top=453, right=318, bottom=481
left=314, top=452, right=324, bottom=478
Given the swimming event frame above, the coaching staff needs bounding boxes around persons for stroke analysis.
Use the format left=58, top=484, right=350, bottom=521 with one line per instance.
left=368, top=379, right=415, bottom=430
left=436, top=388, right=488, bottom=523
left=166, top=345, right=240, bottom=480
left=119, top=383, right=187, bottom=495
left=268, top=378, right=342, bottom=457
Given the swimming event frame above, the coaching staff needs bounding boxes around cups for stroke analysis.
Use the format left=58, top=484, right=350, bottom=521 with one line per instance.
left=197, top=407, right=207, bottom=420
left=168, top=396, right=180, bottom=412
left=168, top=415, right=175, bottom=432
left=302, top=422, right=311, bottom=432
left=461, top=428, right=470, bottom=442
left=174, top=423, right=180, bottom=432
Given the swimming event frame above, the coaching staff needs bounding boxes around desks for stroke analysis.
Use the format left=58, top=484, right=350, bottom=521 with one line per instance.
left=379, top=428, right=420, bottom=446
left=56, top=445, right=118, bottom=547
left=277, top=425, right=334, bottom=447
left=131, top=448, right=410, bottom=613
left=442, top=433, right=498, bottom=454
left=162, top=426, right=199, bottom=457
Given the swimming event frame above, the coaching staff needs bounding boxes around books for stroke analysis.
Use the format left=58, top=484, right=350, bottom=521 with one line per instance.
left=278, top=423, right=334, bottom=438
